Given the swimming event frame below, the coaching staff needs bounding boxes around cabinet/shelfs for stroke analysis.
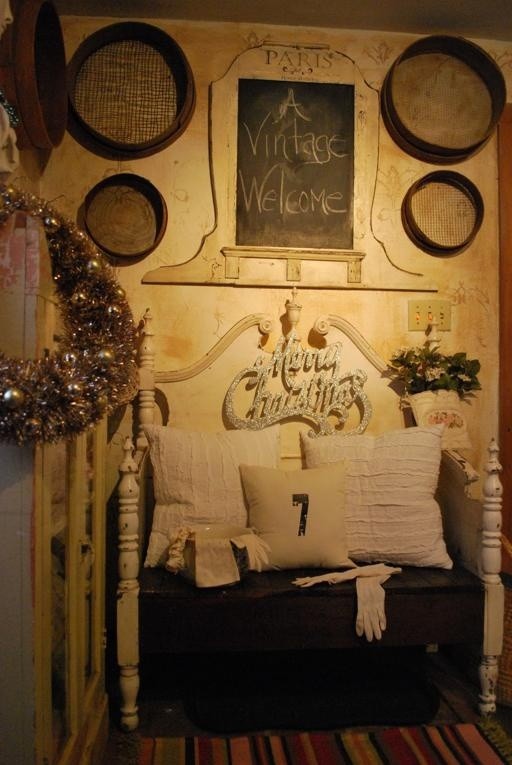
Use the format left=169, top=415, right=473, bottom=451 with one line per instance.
left=0, top=210, right=109, bottom=765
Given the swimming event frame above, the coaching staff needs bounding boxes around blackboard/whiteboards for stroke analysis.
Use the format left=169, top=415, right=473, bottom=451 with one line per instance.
left=236, top=78, right=355, bottom=250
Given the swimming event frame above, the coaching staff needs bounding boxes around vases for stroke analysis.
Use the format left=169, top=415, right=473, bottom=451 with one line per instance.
left=403, top=389, right=471, bottom=451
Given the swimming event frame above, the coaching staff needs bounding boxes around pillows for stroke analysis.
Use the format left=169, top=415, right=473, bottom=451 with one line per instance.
left=140, top=423, right=282, bottom=568
left=239, top=462, right=359, bottom=570
left=296, top=425, right=451, bottom=569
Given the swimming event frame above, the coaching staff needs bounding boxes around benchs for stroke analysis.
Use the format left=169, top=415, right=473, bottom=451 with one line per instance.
left=116, top=287, right=504, bottom=733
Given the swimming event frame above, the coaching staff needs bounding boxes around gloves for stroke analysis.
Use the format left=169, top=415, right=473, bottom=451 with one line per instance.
left=292, top=563, right=402, bottom=588
left=356, top=575, right=387, bottom=642
left=230, top=534, right=272, bottom=573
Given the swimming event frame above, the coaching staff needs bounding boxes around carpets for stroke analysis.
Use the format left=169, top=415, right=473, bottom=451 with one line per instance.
left=112, top=715, right=512, bottom=764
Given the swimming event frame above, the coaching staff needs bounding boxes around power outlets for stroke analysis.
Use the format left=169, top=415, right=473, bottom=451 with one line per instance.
left=409, top=300, right=452, bottom=331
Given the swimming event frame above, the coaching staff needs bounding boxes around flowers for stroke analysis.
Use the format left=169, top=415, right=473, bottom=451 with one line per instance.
left=387, top=340, right=482, bottom=406
